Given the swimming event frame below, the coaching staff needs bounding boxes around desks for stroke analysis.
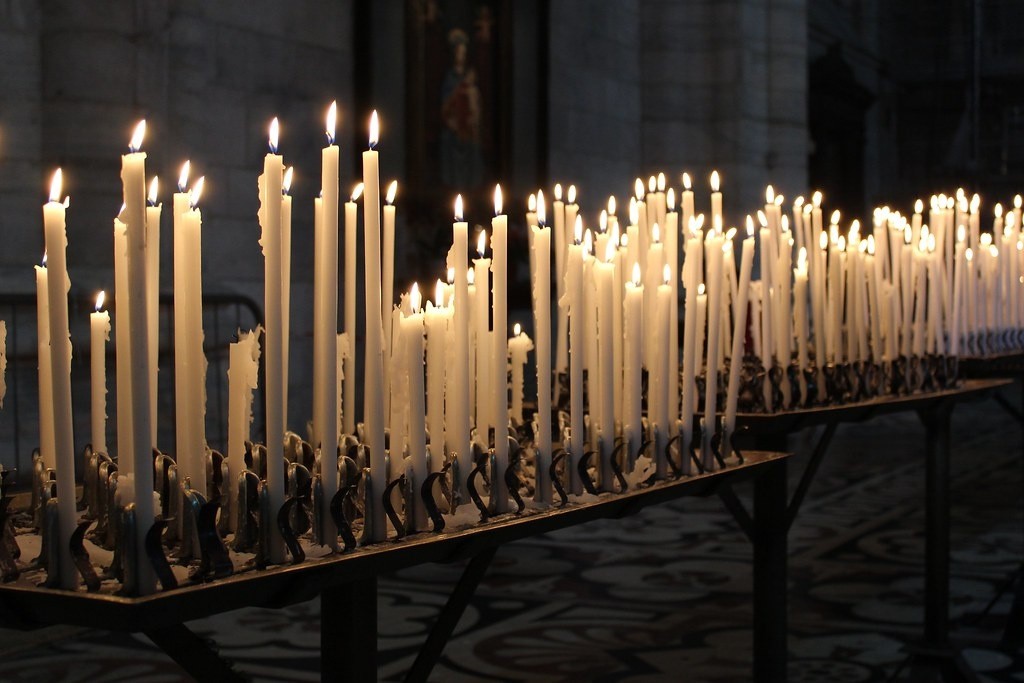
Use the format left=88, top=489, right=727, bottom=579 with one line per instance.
left=0, top=443, right=792, bottom=683
left=522, top=380, right=1024, bottom=641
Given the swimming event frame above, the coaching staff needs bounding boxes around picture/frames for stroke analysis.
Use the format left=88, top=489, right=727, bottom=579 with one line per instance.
left=404, top=0, right=519, bottom=211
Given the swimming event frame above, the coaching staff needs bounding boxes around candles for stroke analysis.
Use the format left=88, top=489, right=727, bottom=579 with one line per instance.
left=0, top=100, right=1024, bottom=588
